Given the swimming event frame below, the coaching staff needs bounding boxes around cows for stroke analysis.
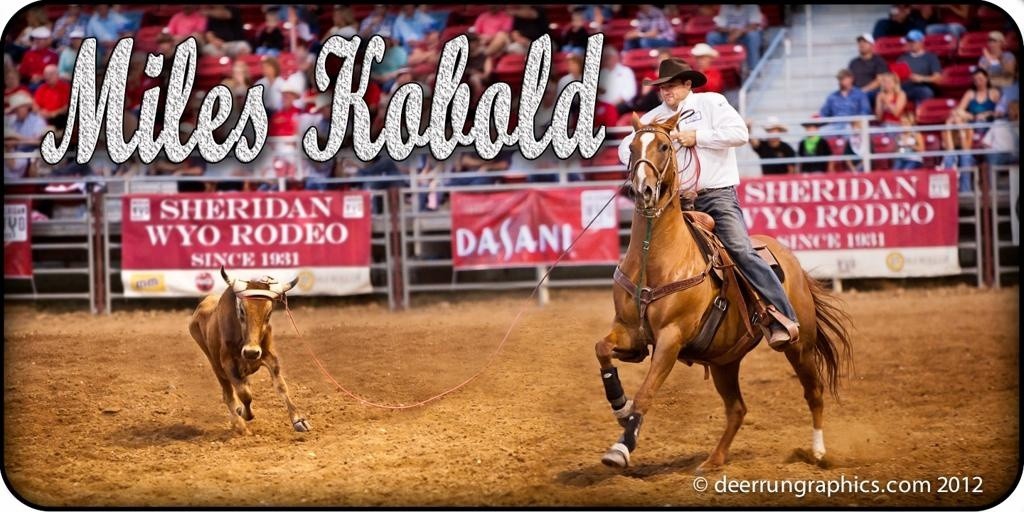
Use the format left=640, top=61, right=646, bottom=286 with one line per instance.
left=185, top=266, right=316, bottom=439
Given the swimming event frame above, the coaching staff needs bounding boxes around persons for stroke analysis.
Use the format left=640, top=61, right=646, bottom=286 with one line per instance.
left=796, top=8, right=1006, bottom=192
left=0, top=0, right=763, bottom=223
left=746, top=116, right=795, bottom=174
left=616, top=58, right=801, bottom=350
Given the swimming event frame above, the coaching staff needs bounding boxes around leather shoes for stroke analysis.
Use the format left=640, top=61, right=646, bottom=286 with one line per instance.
left=768, top=321, right=800, bottom=349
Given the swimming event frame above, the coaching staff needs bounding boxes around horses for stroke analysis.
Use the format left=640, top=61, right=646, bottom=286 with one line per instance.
left=592, top=104, right=855, bottom=475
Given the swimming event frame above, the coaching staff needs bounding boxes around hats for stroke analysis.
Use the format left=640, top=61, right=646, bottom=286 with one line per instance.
left=686, top=43, right=719, bottom=59
left=903, top=29, right=926, bottom=43
left=833, top=70, right=853, bottom=80
left=642, top=58, right=707, bottom=89
left=764, top=123, right=789, bottom=132
left=856, top=33, right=874, bottom=45
